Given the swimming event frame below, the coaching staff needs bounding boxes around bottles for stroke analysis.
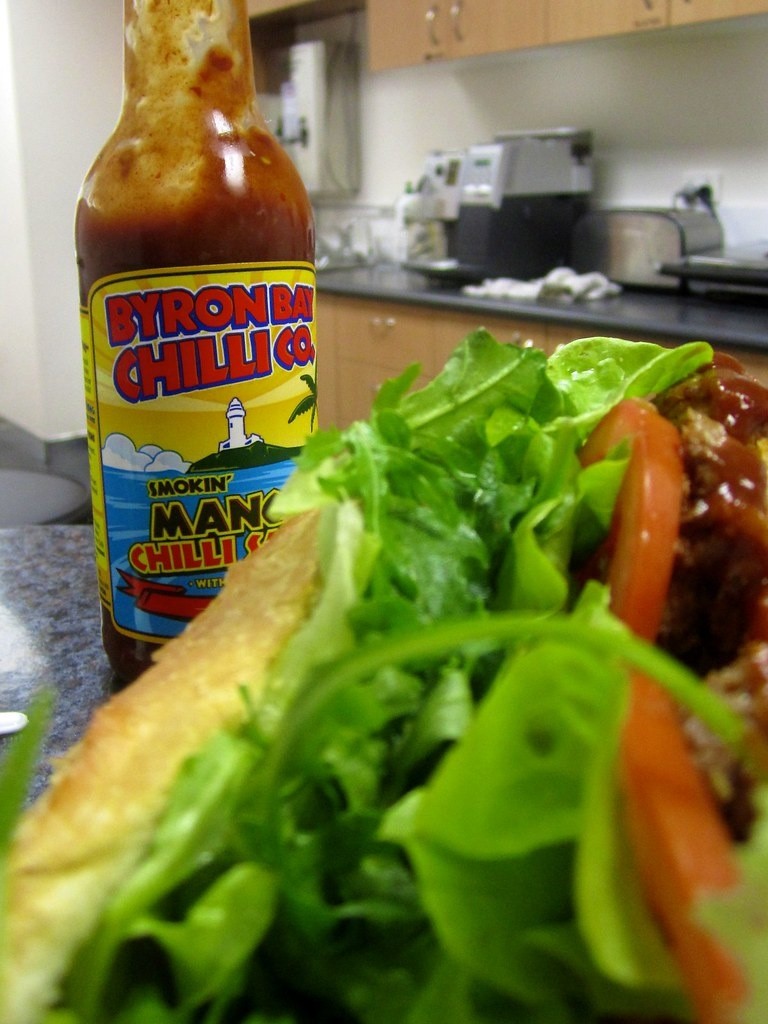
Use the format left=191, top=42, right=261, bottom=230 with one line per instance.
left=76, top=0, right=319, bottom=669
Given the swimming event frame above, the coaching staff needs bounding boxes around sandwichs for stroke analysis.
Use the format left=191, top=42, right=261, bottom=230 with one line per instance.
left=0, top=329, right=767, bottom=1024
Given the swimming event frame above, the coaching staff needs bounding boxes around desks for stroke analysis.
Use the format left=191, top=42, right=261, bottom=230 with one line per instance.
left=2, top=524, right=128, bottom=851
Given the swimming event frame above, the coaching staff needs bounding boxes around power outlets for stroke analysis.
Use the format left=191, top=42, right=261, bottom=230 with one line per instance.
left=682, top=171, right=720, bottom=205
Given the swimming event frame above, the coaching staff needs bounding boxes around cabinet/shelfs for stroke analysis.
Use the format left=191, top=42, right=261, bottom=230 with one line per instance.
left=549, top=1, right=768, bottom=45
left=362, top=2, right=556, bottom=69
left=248, top=1, right=361, bottom=33
left=315, top=292, right=768, bottom=477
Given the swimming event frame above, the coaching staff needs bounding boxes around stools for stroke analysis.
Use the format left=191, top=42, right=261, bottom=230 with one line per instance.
left=2, top=463, right=94, bottom=525
